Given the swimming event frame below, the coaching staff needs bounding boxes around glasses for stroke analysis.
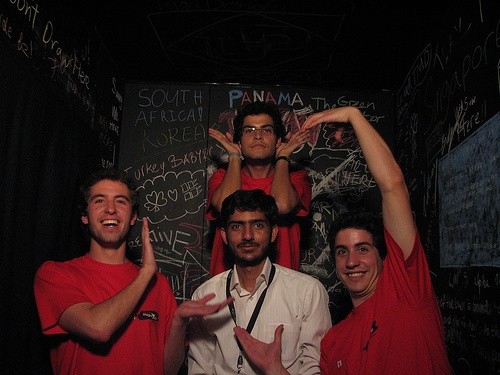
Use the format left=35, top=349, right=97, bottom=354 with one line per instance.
left=241, top=127, right=273, bottom=136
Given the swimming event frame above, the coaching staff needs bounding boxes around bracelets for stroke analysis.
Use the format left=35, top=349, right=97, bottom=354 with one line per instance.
left=272, top=156, right=291, bottom=167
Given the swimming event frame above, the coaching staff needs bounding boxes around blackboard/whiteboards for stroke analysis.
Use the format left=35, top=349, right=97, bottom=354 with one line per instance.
left=0, top=0, right=500, bottom=375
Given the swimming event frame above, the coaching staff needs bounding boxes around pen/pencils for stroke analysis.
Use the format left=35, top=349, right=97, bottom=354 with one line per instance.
left=371, top=320, right=377, bottom=333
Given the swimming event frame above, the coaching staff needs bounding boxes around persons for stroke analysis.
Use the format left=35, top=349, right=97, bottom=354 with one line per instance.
left=188, top=189, right=333, bottom=375
left=232, top=106, right=454, bottom=375
left=208, top=102, right=312, bottom=275
left=33, top=168, right=235, bottom=375
